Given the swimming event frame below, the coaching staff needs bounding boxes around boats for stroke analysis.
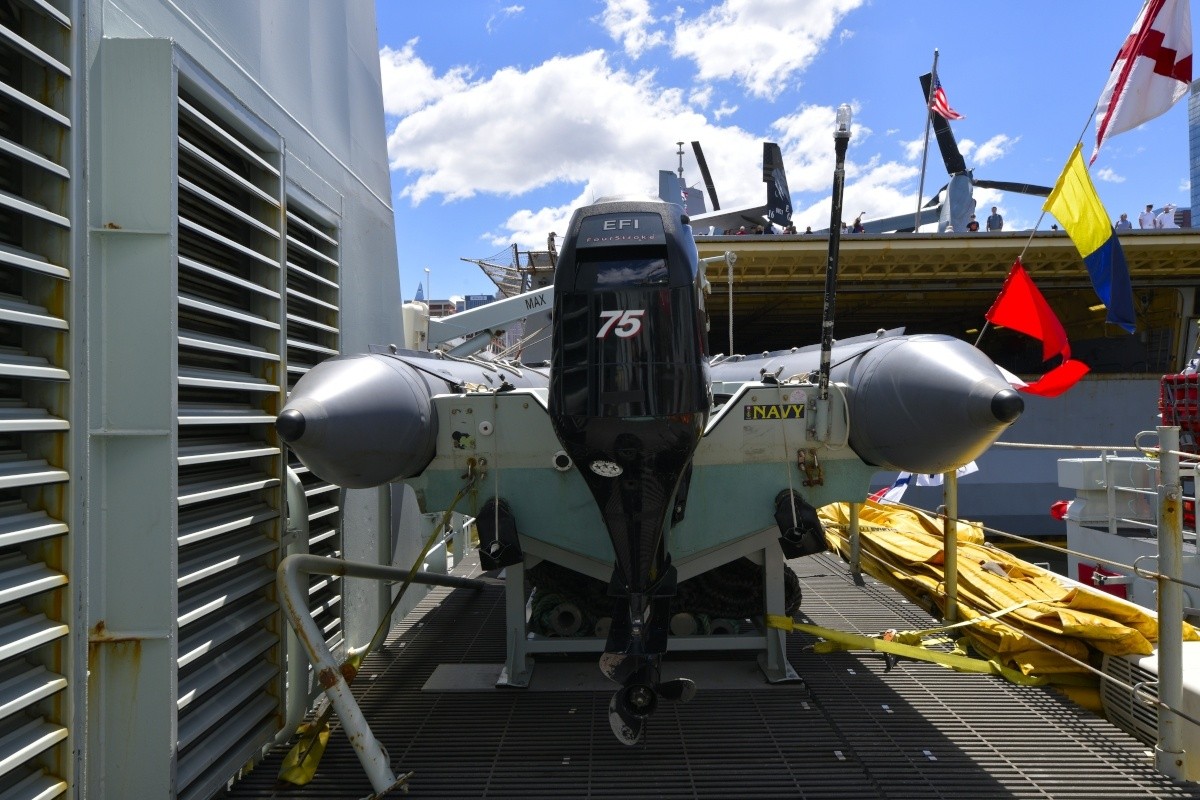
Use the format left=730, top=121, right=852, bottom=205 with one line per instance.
left=459, top=0, right=1199, bottom=544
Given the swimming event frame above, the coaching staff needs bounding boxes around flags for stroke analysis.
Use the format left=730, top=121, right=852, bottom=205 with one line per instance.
left=927, top=69, right=967, bottom=120
left=985, top=0, right=1192, bottom=396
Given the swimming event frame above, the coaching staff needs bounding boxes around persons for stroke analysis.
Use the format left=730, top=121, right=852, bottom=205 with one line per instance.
left=1156, top=204, right=1180, bottom=228
left=1139, top=204, right=1156, bottom=229
left=1115, top=213, right=1132, bottom=230
left=736, top=221, right=813, bottom=235
left=841, top=219, right=864, bottom=234
left=966, top=214, right=979, bottom=232
left=987, top=207, right=1003, bottom=232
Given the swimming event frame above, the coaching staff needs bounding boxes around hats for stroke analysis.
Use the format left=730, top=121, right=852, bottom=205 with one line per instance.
left=1164, top=204, right=1169, bottom=209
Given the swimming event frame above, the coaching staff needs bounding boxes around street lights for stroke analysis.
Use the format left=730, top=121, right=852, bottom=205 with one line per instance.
left=424, top=268, right=431, bottom=307
